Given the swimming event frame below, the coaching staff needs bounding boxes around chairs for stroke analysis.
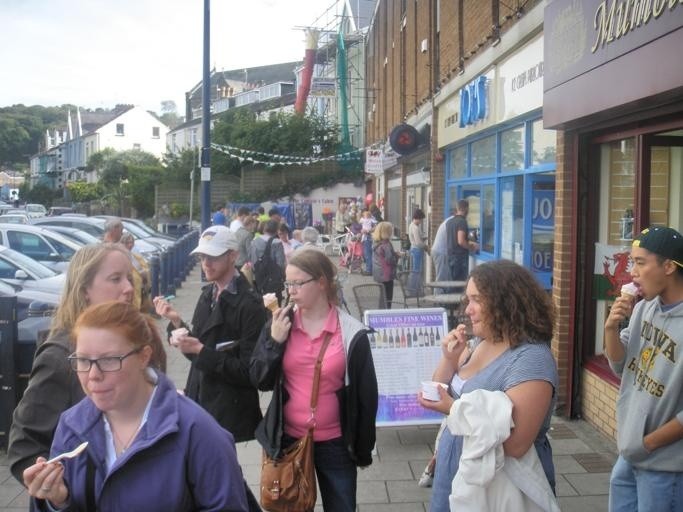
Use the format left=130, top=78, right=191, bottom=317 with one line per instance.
left=397, top=269, right=424, bottom=310
left=318, top=234, right=347, bottom=258
left=353, top=283, right=408, bottom=323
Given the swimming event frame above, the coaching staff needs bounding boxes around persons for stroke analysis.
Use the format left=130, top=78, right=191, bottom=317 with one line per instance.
left=20, top=301, right=247, bottom=511
left=417, top=341, right=481, bottom=489
left=213, top=204, right=320, bottom=307
left=152, top=223, right=268, bottom=442
left=407, top=208, right=429, bottom=297
left=336, top=194, right=384, bottom=276
left=420, top=259, right=558, bottom=511
left=250, top=251, right=378, bottom=511
left=430, top=200, right=479, bottom=294
left=9, top=244, right=167, bottom=484
left=104, top=218, right=162, bottom=320
left=601, top=225, right=681, bottom=512
left=371, top=223, right=406, bottom=310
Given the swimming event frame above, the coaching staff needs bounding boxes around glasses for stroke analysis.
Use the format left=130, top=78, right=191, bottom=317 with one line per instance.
left=195, top=253, right=222, bottom=263
left=67, top=342, right=149, bottom=373
left=283, top=276, right=323, bottom=290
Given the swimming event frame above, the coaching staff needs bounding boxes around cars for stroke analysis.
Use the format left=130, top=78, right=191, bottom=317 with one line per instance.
left=0, top=245, right=68, bottom=296
left=0, top=278, right=63, bottom=323
left=0, top=201, right=181, bottom=273
left=33, top=225, right=151, bottom=295
left=0, top=223, right=85, bottom=273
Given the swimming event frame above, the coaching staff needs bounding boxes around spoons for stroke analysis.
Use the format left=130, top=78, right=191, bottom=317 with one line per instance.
left=41, top=440, right=90, bottom=466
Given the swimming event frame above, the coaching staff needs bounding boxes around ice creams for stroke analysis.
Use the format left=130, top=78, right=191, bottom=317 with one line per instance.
left=262, top=292, right=281, bottom=316
left=621, top=282, right=639, bottom=310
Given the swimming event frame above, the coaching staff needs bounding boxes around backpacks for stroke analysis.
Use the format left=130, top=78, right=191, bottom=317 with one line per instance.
left=253, top=234, right=285, bottom=295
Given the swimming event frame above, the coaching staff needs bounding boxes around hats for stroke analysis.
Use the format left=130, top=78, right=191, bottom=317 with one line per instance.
left=413, top=208, right=426, bottom=219
left=188, top=225, right=241, bottom=257
left=631, top=224, right=683, bottom=269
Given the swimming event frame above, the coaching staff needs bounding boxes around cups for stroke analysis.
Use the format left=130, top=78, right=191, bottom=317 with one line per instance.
left=618, top=217, right=635, bottom=241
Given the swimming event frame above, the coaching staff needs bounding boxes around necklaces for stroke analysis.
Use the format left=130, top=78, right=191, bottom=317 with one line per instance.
left=111, top=424, right=140, bottom=455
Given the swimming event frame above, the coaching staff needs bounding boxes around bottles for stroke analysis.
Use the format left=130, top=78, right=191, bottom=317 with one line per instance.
left=368, top=326, right=441, bottom=350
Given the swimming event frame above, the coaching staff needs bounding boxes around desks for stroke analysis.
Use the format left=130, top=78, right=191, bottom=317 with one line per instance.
left=421, top=294, right=465, bottom=327
left=424, top=281, right=464, bottom=294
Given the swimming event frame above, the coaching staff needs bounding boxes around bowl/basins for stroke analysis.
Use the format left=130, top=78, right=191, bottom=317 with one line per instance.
left=419, top=379, right=450, bottom=401
left=170, top=327, right=187, bottom=344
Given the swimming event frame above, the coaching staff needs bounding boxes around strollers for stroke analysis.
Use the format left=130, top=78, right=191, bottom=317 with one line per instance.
left=339, top=234, right=363, bottom=274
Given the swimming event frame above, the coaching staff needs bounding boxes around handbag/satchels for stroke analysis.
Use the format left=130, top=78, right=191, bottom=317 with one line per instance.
left=259, top=423, right=319, bottom=512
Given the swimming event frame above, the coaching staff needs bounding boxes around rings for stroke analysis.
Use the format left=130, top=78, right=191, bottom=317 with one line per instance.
left=40, top=486, right=52, bottom=491
